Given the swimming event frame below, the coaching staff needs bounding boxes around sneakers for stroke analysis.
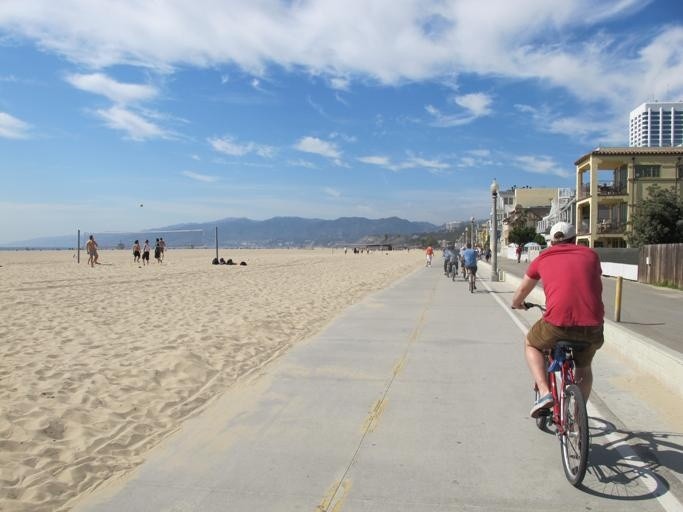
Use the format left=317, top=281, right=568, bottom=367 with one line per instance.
left=531, top=393, right=555, bottom=419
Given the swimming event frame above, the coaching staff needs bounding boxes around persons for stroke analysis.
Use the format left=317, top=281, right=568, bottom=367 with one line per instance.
left=86, top=235, right=98, bottom=266
left=425, top=244, right=434, bottom=267
left=87, top=256, right=95, bottom=264
left=443, top=243, right=490, bottom=290
left=511, top=221, right=604, bottom=435
left=344, top=247, right=370, bottom=254
left=516, top=245, right=523, bottom=262
left=133, top=238, right=166, bottom=265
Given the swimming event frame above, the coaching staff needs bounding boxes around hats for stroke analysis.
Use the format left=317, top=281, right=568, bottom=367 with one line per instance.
left=550, top=221, right=576, bottom=242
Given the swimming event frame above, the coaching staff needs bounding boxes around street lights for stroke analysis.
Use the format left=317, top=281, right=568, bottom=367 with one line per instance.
left=489, top=177, right=500, bottom=281
left=456, top=215, right=476, bottom=252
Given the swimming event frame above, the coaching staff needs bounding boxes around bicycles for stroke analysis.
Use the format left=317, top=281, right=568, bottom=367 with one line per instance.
left=511, top=301, right=591, bottom=489
left=441, top=258, right=477, bottom=293
